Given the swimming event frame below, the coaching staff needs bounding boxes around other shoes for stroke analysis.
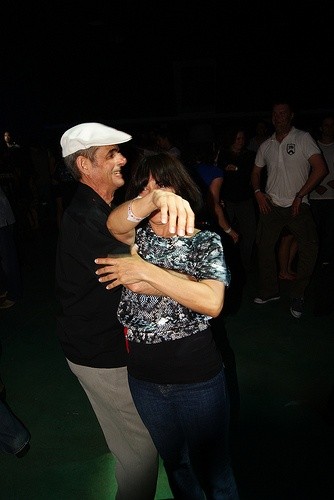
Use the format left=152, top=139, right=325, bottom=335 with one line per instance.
left=0, top=291, right=16, bottom=308
left=254, top=290, right=281, bottom=303
left=290, top=295, right=303, bottom=318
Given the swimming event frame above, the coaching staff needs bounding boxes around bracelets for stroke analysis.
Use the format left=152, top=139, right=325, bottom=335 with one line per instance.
left=296, top=194, right=303, bottom=199
left=225, top=227, right=232, bottom=233
left=128, top=198, right=150, bottom=222
left=254, top=189, right=260, bottom=195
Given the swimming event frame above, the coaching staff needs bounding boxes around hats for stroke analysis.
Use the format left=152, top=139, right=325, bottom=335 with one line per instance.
left=60, top=123, right=133, bottom=158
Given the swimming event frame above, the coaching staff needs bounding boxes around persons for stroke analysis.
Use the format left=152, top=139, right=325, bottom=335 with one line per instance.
left=151, top=99, right=334, bottom=318
left=0, top=128, right=68, bottom=230
left=52, top=122, right=198, bottom=500
left=94, top=154, right=239, bottom=500
left=0, top=401, right=31, bottom=458
left=0, top=188, right=24, bottom=308
